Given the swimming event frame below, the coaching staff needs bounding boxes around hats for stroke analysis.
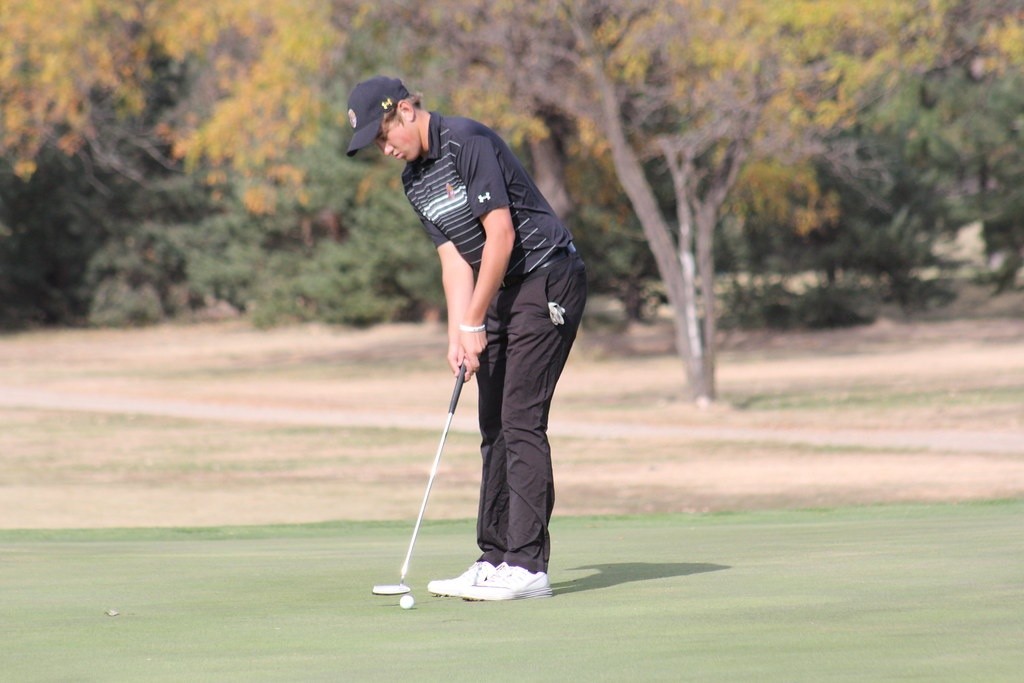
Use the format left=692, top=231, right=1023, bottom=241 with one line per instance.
left=339, top=75, right=412, bottom=156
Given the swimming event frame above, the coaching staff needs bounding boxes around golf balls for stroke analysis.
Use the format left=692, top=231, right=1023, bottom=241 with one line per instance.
left=400, top=595, right=415, bottom=609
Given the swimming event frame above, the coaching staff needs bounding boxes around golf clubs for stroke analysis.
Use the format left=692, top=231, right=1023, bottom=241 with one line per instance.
left=372, top=356, right=467, bottom=595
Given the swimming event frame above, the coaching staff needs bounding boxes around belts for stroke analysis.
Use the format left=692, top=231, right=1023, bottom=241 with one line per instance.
left=533, top=239, right=578, bottom=270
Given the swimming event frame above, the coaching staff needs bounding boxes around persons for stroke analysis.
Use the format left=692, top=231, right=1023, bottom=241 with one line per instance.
left=345, top=74, right=586, bottom=600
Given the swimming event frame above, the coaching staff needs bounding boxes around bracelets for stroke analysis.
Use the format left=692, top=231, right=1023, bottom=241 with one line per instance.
left=460, top=326, right=486, bottom=332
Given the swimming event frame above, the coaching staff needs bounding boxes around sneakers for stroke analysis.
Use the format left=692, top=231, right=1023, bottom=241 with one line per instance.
left=463, top=563, right=554, bottom=603
left=428, top=563, right=495, bottom=596
